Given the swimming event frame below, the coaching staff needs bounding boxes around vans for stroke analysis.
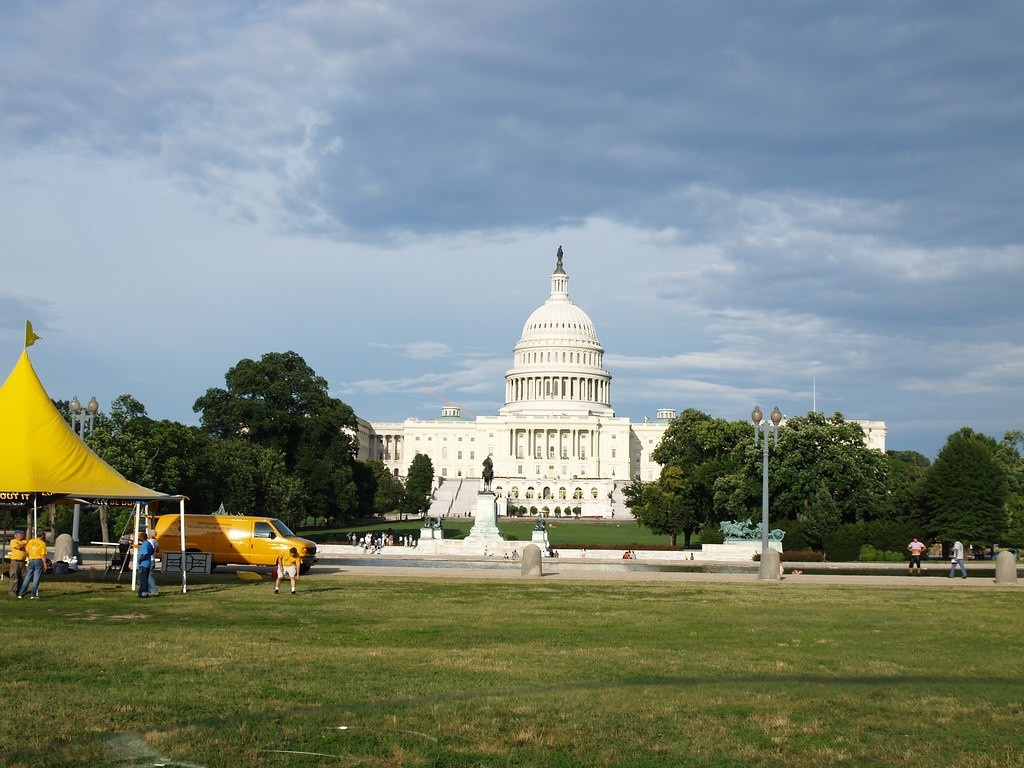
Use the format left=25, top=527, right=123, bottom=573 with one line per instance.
left=132, top=512, right=318, bottom=576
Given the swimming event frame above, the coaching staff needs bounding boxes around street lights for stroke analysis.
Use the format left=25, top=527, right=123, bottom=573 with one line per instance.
left=751, top=405, right=783, bottom=549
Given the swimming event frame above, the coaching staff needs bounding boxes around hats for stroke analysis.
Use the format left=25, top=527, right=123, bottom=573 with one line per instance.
left=148, top=530, right=157, bottom=538
left=14, top=530, right=25, bottom=536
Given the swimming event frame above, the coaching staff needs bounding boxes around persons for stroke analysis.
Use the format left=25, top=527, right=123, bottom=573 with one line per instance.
left=541, top=548, right=559, bottom=559
left=622, top=550, right=635, bottom=560
left=905, top=533, right=924, bottom=575
left=18, top=528, right=47, bottom=601
left=504, top=549, right=519, bottom=560
left=138, top=530, right=162, bottom=598
left=273, top=546, right=301, bottom=594
left=9, top=530, right=28, bottom=595
left=949, top=538, right=967, bottom=579
left=352, top=531, right=416, bottom=555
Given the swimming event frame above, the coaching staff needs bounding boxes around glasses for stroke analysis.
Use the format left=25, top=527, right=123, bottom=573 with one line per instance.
left=19, top=532, right=24, bottom=535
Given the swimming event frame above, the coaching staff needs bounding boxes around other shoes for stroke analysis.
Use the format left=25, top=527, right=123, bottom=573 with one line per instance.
left=18, top=596, right=23, bottom=599
left=30, top=596, right=39, bottom=600
left=292, top=591, right=296, bottom=595
left=274, top=590, right=279, bottom=594
left=149, top=590, right=158, bottom=596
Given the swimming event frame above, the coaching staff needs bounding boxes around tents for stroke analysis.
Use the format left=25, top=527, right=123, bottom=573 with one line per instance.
left=0, top=321, right=189, bottom=593
